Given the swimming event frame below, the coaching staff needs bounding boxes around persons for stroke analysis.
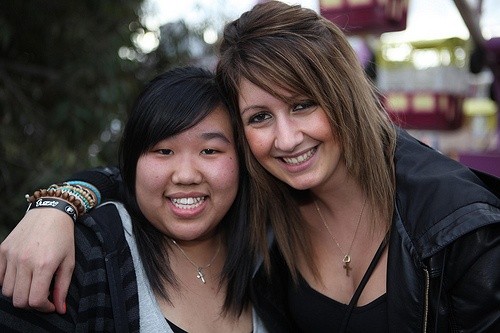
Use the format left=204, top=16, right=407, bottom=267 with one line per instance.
left=0, top=0, right=500, bottom=333
left=0, top=66, right=270, bottom=333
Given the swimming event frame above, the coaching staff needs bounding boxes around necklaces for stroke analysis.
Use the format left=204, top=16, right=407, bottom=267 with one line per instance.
left=171, top=239, right=222, bottom=284
left=315, top=197, right=368, bottom=277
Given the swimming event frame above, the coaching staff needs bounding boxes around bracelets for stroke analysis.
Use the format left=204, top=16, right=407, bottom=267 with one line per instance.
left=25, top=180, right=100, bottom=220
